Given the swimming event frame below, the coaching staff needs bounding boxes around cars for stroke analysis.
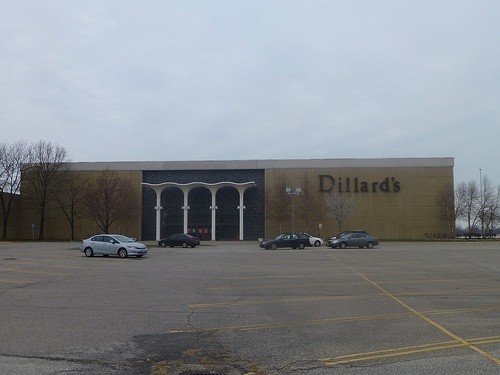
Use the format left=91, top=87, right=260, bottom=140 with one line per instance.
left=329, top=232, right=379, bottom=249
left=157, top=233, right=201, bottom=248
left=80, top=234, right=149, bottom=259
left=326, top=230, right=369, bottom=247
left=299, top=233, right=324, bottom=247
left=258, top=232, right=312, bottom=250
